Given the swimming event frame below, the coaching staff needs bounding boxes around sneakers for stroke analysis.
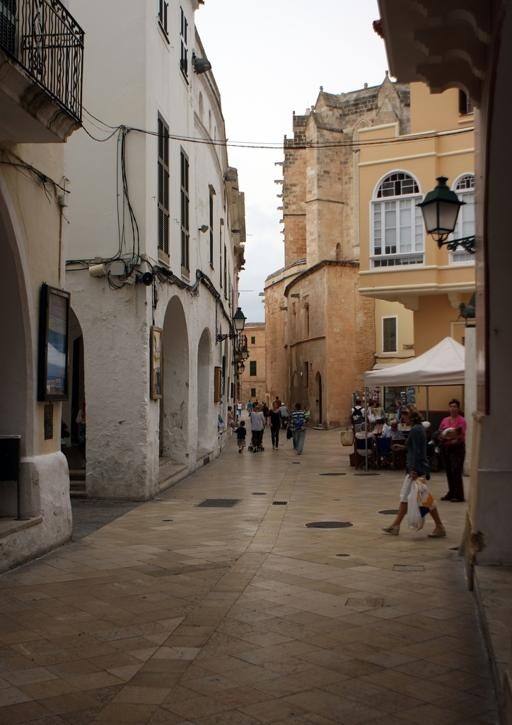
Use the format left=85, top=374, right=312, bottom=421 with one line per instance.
left=428, top=530, right=446, bottom=538
left=383, top=526, right=400, bottom=535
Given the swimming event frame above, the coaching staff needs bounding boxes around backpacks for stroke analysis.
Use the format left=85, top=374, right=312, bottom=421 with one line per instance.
left=351, top=407, right=364, bottom=424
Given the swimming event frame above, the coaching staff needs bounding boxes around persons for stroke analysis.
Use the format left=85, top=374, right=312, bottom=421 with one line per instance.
left=428, top=427, right=462, bottom=453
left=438, top=398, right=466, bottom=502
left=351, top=398, right=406, bottom=469
left=381, top=403, right=446, bottom=537
left=227, top=396, right=310, bottom=455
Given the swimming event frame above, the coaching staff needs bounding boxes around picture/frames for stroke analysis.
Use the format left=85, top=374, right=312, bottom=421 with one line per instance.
left=148, top=326, right=164, bottom=402
left=36, top=282, right=71, bottom=403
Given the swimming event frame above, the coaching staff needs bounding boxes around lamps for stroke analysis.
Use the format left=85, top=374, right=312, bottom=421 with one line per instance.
left=234, top=364, right=245, bottom=377
left=414, top=176, right=476, bottom=256
left=233, top=341, right=249, bottom=363
left=215, top=307, right=247, bottom=343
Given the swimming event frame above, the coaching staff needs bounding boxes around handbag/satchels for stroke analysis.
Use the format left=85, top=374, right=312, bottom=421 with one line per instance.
left=415, top=477, right=434, bottom=517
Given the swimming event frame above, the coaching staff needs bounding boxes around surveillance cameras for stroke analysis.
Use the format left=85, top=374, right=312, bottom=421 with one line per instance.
left=142, top=271, right=153, bottom=286
